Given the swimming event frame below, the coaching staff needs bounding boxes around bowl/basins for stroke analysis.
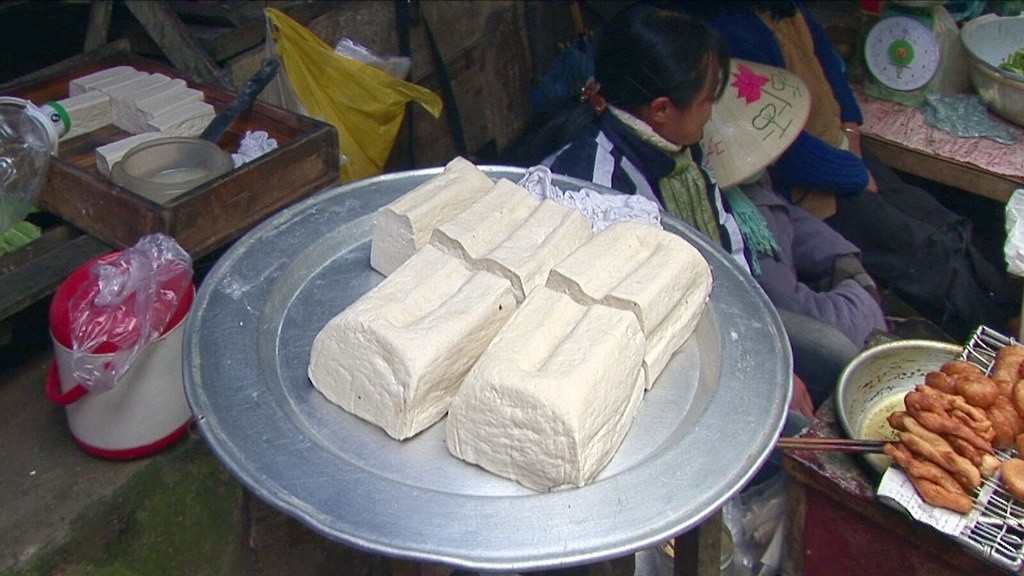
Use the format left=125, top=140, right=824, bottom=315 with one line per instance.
left=836, top=340, right=997, bottom=485
left=961, top=10, right=1024, bottom=127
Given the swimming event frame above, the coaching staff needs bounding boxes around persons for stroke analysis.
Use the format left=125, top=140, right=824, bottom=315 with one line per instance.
left=550, top=0, right=1024, bottom=476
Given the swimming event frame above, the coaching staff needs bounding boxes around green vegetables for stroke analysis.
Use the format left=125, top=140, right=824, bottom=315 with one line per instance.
left=0, top=193, right=41, bottom=260
left=999, top=48, right=1024, bottom=74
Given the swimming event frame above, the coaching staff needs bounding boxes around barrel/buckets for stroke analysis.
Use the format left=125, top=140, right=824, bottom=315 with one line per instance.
left=45, top=250, right=196, bottom=459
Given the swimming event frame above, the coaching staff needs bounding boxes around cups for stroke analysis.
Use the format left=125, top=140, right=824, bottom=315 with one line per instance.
left=110, top=136, right=234, bottom=205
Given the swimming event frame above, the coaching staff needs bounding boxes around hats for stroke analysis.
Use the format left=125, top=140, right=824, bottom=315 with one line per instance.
left=698, top=58, right=810, bottom=189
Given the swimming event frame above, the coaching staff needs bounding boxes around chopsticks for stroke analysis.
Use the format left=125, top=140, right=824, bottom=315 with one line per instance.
left=778, top=438, right=896, bottom=454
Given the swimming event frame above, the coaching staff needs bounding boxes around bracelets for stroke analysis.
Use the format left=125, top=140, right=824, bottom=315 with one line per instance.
left=841, top=124, right=861, bottom=137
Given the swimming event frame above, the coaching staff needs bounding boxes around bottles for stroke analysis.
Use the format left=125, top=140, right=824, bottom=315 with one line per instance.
left=0, top=96, right=71, bottom=234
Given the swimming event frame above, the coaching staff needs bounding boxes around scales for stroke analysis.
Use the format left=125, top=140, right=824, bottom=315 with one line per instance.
left=859, top=0, right=961, bottom=109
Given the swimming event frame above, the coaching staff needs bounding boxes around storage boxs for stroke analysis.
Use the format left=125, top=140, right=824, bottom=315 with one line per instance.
left=0, top=38, right=343, bottom=263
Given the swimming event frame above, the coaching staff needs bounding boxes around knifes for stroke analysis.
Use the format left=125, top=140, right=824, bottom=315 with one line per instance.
left=199, top=57, right=281, bottom=143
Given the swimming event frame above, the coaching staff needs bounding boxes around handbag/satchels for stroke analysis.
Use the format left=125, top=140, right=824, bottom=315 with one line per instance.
left=265, top=8, right=442, bottom=184
